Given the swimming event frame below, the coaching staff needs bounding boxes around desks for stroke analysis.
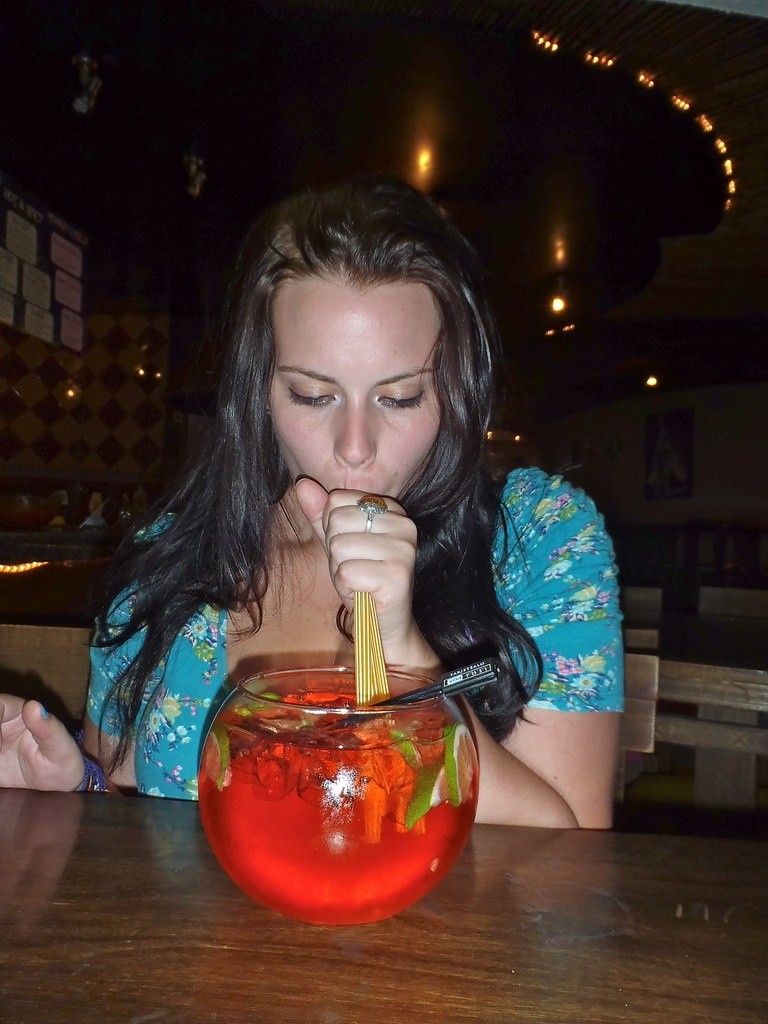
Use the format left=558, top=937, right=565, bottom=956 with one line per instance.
left=2, top=787, right=767, bottom=1022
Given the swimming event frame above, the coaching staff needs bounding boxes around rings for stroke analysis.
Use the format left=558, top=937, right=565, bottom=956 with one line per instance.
left=357, top=494, right=389, bottom=533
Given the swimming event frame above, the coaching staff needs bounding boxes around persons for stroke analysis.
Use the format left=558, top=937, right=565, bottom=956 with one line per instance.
left=0, top=174, right=626, bottom=832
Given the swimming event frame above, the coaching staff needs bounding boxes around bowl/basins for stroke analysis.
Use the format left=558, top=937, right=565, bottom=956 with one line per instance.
left=0, top=494, right=62, bottom=529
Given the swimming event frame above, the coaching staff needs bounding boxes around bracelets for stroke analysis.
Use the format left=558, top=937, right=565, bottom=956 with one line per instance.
left=73, top=729, right=105, bottom=792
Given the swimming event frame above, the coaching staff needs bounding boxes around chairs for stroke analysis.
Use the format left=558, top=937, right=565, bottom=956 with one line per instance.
left=2, top=528, right=768, bottom=837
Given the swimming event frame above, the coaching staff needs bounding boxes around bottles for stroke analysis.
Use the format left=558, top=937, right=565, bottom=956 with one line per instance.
left=132, top=486, right=146, bottom=513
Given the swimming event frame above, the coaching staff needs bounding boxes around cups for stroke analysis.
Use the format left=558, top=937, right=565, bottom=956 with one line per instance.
left=198, top=666, right=481, bottom=926
